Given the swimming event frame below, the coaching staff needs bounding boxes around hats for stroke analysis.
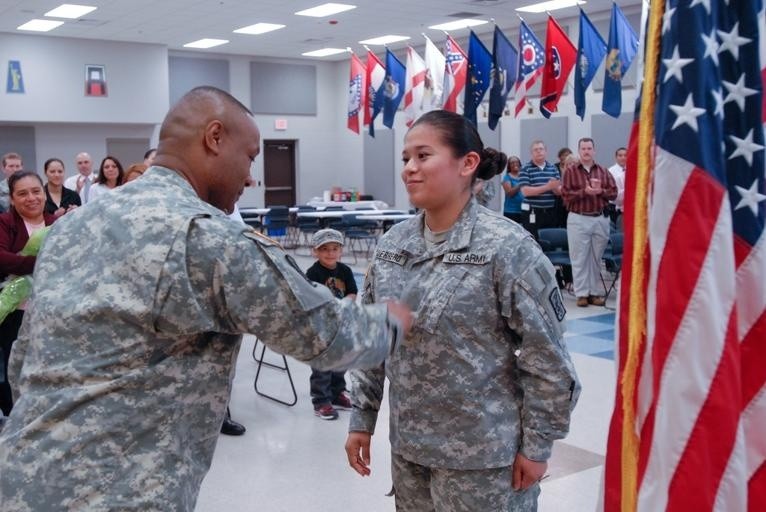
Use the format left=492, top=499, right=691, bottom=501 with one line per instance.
left=310, top=227, right=345, bottom=251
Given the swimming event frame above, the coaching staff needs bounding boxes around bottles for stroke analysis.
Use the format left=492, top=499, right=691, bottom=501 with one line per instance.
left=323, top=185, right=360, bottom=202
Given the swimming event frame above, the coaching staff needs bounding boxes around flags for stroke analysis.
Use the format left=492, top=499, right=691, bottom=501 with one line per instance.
left=595, top=0, right=766, bottom=512
left=346, top=0, right=641, bottom=140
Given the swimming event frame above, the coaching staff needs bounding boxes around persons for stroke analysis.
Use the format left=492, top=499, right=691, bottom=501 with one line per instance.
left=304, top=110, right=629, bottom=512
left=2, top=84, right=413, bottom=510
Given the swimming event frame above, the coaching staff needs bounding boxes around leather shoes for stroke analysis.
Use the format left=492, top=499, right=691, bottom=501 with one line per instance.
left=220, top=416, right=246, bottom=436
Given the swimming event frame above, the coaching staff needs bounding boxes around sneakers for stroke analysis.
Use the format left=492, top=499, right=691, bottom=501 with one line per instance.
left=330, top=392, right=353, bottom=411
left=576, top=296, right=589, bottom=306
left=589, top=294, right=606, bottom=306
left=314, top=404, right=339, bottom=420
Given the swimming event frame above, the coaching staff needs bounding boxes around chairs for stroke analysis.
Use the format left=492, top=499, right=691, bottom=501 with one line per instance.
left=601, top=233, right=622, bottom=311
left=240, top=205, right=415, bottom=265
left=537, top=228, right=574, bottom=297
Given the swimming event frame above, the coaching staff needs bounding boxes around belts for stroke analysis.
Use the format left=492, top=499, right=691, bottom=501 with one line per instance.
left=570, top=209, right=604, bottom=217
